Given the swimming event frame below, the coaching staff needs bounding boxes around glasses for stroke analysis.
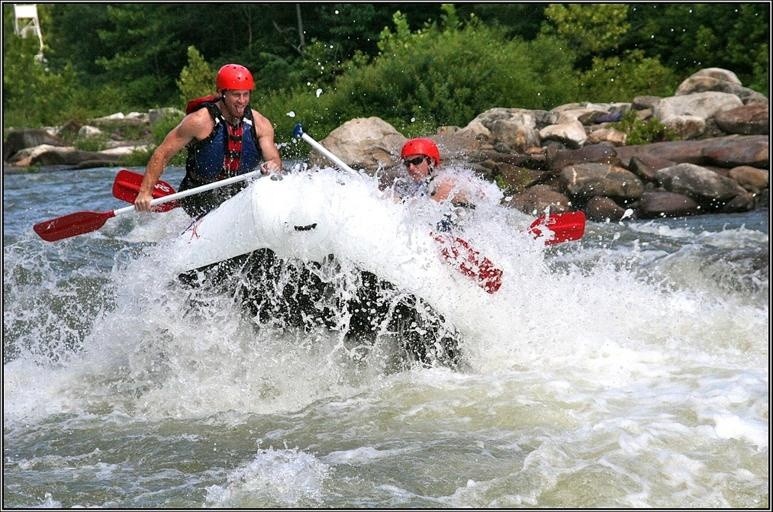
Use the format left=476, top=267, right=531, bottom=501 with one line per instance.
left=402, top=157, right=423, bottom=168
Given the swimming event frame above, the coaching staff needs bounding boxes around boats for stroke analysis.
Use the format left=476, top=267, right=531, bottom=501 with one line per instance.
left=170, top=161, right=481, bottom=377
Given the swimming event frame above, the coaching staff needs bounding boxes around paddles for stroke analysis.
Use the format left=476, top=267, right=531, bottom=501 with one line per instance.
left=111, top=167, right=182, bottom=213
left=525, top=210, right=586, bottom=245
left=291, top=124, right=503, bottom=296
left=33, top=170, right=259, bottom=242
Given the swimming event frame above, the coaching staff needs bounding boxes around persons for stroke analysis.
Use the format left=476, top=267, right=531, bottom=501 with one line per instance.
left=133, top=64, right=283, bottom=220
left=383, top=138, right=475, bottom=231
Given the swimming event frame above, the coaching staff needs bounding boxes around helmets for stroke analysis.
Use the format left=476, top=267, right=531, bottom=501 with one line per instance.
left=214, top=63, right=254, bottom=91
left=398, top=137, right=440, bottom=166
